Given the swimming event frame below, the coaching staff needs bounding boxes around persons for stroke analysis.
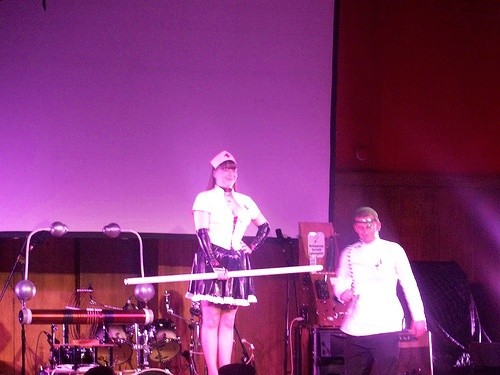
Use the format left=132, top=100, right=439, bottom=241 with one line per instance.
left=333, top=206, right=429, bottom=375
left=184, top=150, right=272, bottom=375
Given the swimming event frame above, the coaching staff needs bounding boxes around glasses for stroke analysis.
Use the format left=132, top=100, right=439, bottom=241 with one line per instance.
left=355, top=218, right=376, bottom=226
left=216, top=166, right=237, bottom=171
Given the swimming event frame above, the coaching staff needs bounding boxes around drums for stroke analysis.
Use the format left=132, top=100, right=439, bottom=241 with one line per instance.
left=52, top=344, right=95, bottom=373
left=95, top=324, right=135, bottom=367
left=141, top=318, right=182, bottom=364
